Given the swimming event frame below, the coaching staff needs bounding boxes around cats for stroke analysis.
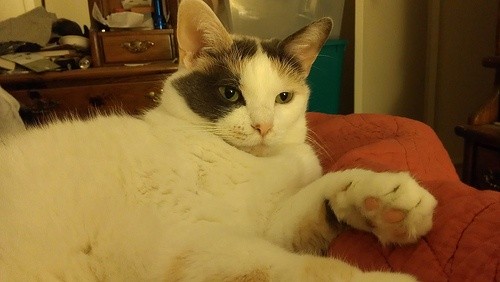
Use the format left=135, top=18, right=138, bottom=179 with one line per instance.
left=0, top=0, right=438, bottom=282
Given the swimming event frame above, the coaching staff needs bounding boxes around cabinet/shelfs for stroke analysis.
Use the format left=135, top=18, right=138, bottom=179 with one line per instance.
left=0, top=28, right=178, bottom=126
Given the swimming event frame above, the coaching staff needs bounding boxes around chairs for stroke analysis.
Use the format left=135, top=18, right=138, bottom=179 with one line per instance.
left=451, top=57, right=500, bottom=189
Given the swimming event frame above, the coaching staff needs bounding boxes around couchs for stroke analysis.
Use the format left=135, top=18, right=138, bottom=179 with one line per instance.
left=294, top=113, right=500, bottom=282
